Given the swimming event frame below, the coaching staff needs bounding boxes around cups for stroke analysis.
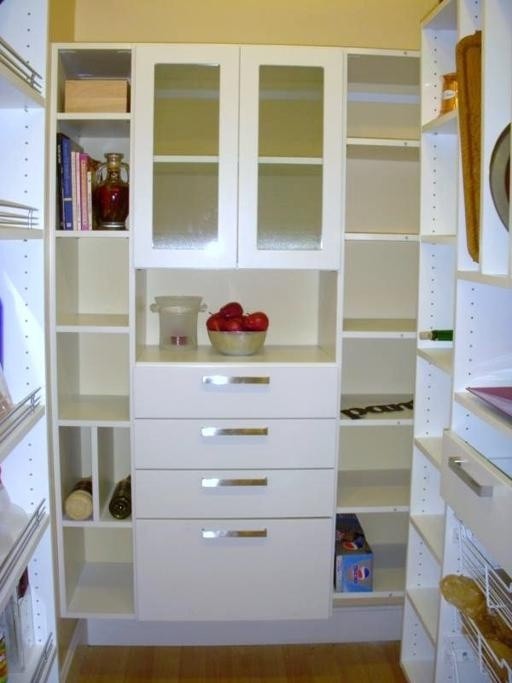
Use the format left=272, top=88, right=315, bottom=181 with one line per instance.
left=207, top=328, right=267, bottom=356
left=151, top=295, right=206, bottom=354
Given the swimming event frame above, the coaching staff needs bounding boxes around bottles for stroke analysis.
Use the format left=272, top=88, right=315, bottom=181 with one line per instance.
left=63, top=474, right=94, bottom=520
left=111, top=475, right=131, bottom=521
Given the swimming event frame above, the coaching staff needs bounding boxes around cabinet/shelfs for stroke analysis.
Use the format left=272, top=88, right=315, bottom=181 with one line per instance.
left=50, top=46, right=132, bottom=620
left=338, top=51, right=421, bottom=608
left=434, top=0, right=512, bottom=677
left=132, top=363, right=328, bottom=622
left=130, top=47, right=339, bottom=268
left=398, top=13, right=454, bottom=678
left=0, top=1, right=55, bottom=683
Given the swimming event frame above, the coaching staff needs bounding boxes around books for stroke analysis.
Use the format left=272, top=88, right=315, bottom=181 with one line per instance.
left=56, top=132, right=104, bottom=231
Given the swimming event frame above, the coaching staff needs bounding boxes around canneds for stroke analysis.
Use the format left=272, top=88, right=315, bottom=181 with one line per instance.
left=63, top=479, right=93, bottom=520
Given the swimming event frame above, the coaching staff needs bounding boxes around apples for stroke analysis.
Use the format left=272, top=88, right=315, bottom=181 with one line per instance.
left=207, top=302, right=269, bottom=332
